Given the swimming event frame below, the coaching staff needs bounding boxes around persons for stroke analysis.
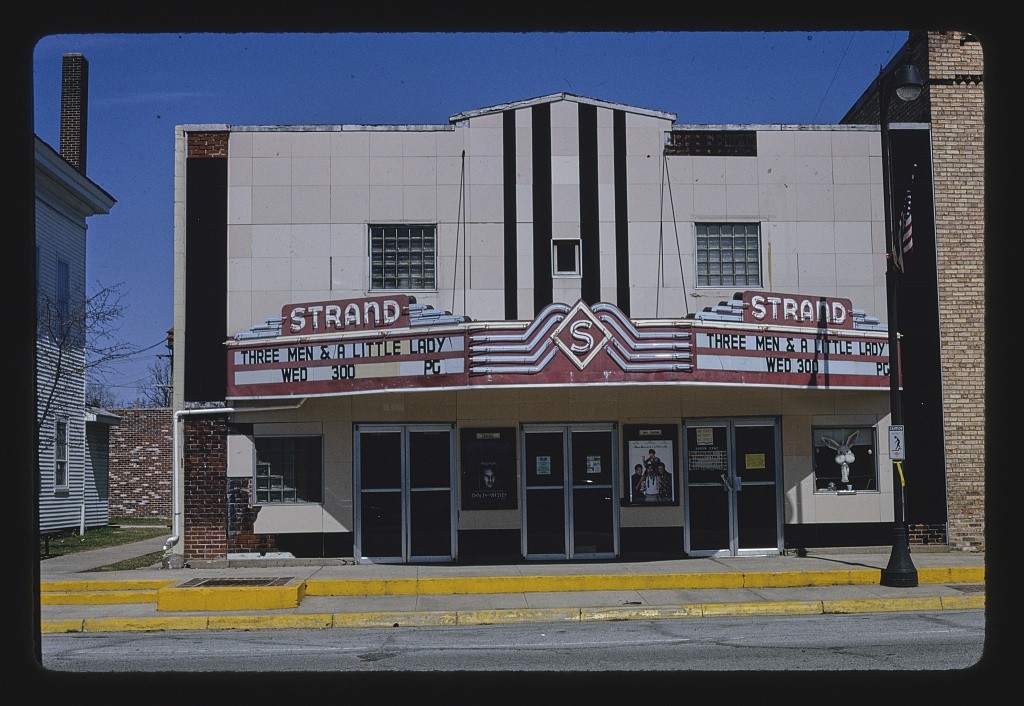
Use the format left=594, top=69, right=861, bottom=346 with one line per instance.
left=632, top=449, right=672, bottom=501
left=483, top=468, right=495, bottom=487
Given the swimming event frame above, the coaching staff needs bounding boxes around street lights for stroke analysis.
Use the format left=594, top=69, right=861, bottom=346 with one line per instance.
left=878, top=63, right=931, bottom=589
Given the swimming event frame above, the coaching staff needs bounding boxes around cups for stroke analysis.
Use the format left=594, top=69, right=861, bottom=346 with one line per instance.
left=846, top=485, right=852, bottom=491
